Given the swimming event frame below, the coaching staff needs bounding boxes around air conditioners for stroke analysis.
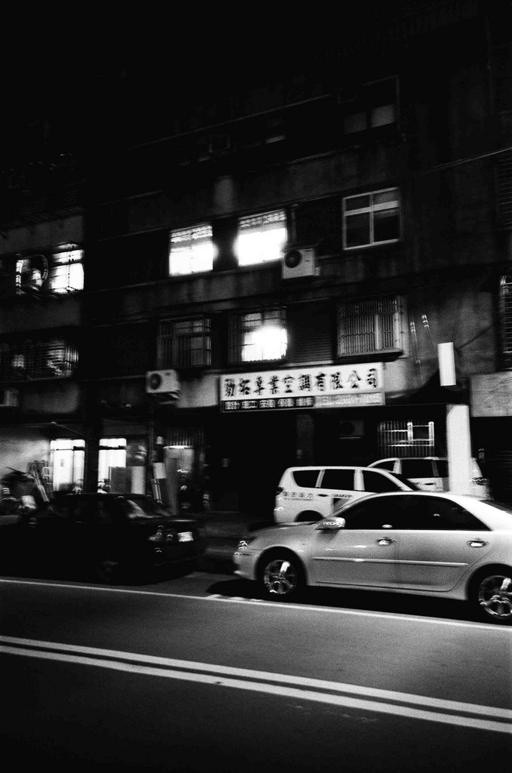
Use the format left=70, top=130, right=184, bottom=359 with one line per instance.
left=282, top=247, right=314, bottom=278
left=145, top=369, right=181, bottom=393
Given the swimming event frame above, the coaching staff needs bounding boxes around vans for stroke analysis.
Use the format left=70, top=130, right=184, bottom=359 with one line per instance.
left=274, top=466, right=423, bottom=525
left=366, top=457, right=490, bottom=499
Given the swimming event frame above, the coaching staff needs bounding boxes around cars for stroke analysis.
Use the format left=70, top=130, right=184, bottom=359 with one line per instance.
left=15, top=493, right=202, bottom=586
left=233, top=492, right=512, bottom=625
